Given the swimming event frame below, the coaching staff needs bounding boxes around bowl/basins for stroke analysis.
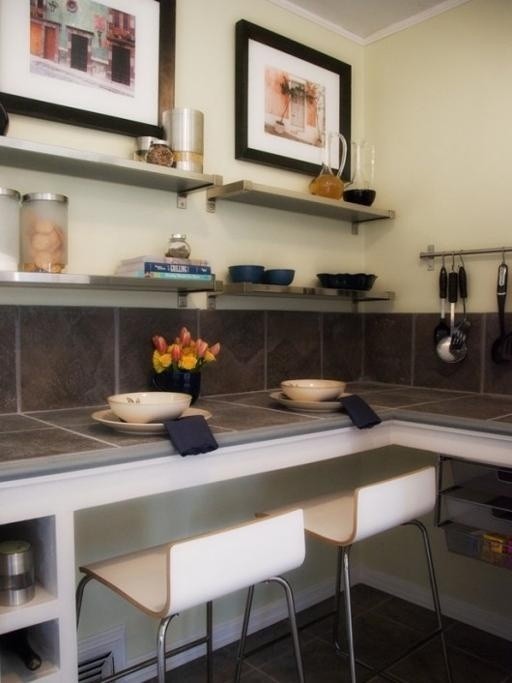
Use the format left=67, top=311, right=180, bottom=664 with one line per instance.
left=228, top=265, right=265, bottom=282
left=281, top=378, right=347, bottom=401
left=317, top=273, right=347, bottom=288
left=346, top=274, right=378, bottom=290
left=266, top=269, right=295, bottom=285
left=107, top=391, right=192, bottom=423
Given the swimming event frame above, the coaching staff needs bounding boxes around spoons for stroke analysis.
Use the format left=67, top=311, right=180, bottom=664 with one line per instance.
left=436, top=254, right=467, bottom=365
left=491, top=265, right=512, bottom=364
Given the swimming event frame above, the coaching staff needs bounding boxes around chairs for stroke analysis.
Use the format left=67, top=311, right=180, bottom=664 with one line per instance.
left=72, top=507, right=305, bottom=677
left=254, top=464, right=453, bottom=681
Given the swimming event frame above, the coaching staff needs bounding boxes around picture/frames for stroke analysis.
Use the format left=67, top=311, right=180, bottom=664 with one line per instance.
left=232, top=18, right=353, bottom=182
left=0, top=2, right=177, bottom=141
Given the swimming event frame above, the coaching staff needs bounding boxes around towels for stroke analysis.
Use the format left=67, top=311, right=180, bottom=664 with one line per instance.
left=161, top=414, right=219, bottom=457
left=343, top=394, right=383, bottom=431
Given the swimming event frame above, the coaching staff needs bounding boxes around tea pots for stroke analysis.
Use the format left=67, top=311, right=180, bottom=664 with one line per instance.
left=308, top=131, right=347, bottom=200
left=342, top=139, right=376, bottom=208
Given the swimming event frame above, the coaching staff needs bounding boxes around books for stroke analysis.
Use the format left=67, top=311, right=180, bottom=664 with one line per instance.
left=116, top=255, right=216, bottom=281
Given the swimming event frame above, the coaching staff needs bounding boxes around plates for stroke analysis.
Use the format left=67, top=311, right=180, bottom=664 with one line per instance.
left=270, top=391, right=352, bottom=412
left=91, top=409, right=212, bottom=436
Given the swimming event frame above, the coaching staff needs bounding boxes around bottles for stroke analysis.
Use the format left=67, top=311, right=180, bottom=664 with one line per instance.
left=134, top=136, right=157, bottom=162
left=0, top=629, right=41, bottom=670
left=0, top=188, right=22, bottom=272
left=22, top=193, right=69, bottom=275
left=163, top=234, right=191, bottom=259
left=146, top=140, right=174, bottom=168
